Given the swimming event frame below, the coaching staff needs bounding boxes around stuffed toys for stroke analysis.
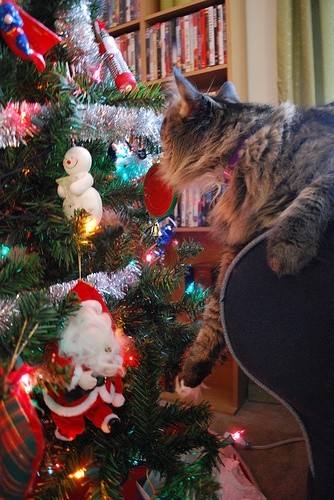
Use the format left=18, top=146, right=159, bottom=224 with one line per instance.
left=42, top=279, right=140, bottom=441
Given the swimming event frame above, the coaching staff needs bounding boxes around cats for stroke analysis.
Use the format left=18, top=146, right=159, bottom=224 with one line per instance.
left=151, top=68, right=334, bottom=388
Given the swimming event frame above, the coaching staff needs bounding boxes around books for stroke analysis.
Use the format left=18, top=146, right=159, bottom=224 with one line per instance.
left=156, top=170, right=232, bottom=307
left=83, top=0, right=232, bottom=82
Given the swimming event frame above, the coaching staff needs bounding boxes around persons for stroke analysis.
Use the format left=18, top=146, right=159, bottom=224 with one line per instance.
left=51, top=146, right=106, bottom=229
